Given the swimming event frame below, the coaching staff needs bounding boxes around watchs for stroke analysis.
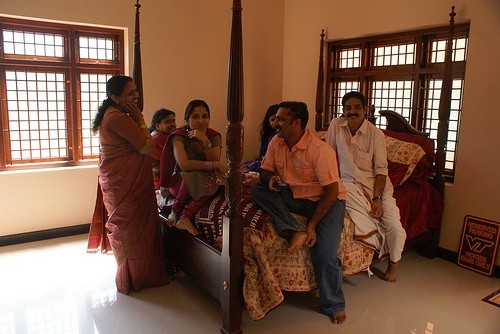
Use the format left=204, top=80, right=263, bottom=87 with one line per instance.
left=203, top=141, right=214, bottom=150
left=373, top=196, right=384, bottom=202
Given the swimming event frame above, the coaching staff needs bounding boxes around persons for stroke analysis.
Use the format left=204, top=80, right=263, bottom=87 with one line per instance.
left=147, top=108, right=178, bottom=191
left=85, top=73, right=171, bottom=295
left=249, top=101, right=348, bottom=325
left=326, top=91, right=408, bottom=283
left=249, top=103, right=284, bottom=170
left=159, top=98, right=229, bottom=236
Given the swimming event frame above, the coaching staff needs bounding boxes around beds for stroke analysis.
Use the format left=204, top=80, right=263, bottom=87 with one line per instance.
left=134, top=0, right=455, bottom=334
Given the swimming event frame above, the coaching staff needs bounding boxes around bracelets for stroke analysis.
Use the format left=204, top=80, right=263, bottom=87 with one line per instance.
left=139, top=124, right=147, bottom=130
left=204, top=161, right=212, bottom=172
